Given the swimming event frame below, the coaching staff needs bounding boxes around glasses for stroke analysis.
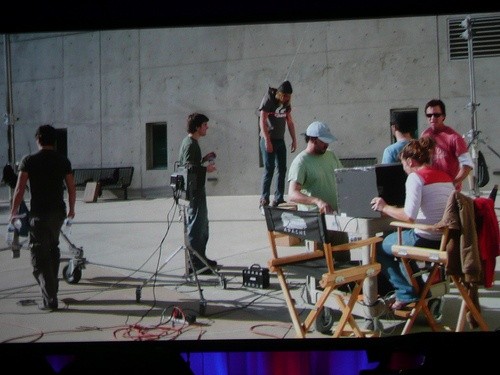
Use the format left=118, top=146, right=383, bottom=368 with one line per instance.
left=425, top=113, right=442, bottom=118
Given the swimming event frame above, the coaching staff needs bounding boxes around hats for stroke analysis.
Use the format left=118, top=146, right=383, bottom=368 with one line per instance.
left=305, top=121, right=338, bottom=143
left=277, top=81, right=292, bottom=93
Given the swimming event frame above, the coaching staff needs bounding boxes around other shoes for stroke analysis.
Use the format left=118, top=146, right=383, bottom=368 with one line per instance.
left=39, top=304, right=57, bottom=313
left=391, top=302, right=411, bottom=310
left=186, top=261, right=216, bottom=274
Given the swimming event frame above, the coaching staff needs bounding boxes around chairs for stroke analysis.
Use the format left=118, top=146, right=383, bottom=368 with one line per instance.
left=389, top=184, right=500, bottom=335
left=263, top=204, right=381, bottom=339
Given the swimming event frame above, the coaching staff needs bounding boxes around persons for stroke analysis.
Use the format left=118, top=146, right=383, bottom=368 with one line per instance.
left=370, top=137, right=457, bottom=309
left=420, top=97, right=475, bottom=193
left=379, top=110, right=418, bottom=164
left=257, top=79, right=297, bottom=216
left=8, top=124, right=76, bottom=312
left=176, top=112, right=217, bottom=276
left=287, top=121, right=360, bottom=269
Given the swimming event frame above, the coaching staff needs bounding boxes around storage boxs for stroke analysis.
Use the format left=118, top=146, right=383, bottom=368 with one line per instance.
left=268, top=202, right=304, bottom=246
left=84, top=181, right=100, bottom=203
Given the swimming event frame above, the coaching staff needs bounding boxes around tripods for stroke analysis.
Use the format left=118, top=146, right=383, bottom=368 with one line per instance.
left=136, top=205, right=227, bottom=315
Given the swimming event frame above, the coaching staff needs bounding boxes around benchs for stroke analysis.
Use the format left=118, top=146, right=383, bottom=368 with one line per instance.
left=63, top=166, right=134, bottom=201
